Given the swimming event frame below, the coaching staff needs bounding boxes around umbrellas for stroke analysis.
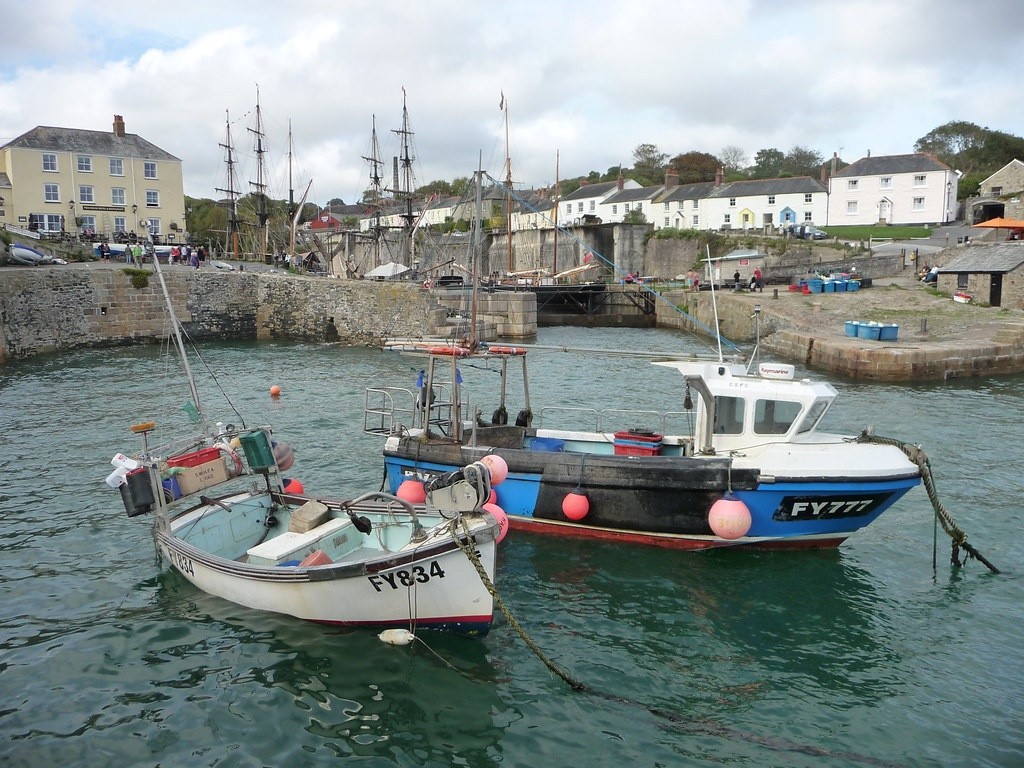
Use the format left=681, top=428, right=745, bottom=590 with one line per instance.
left=971, top=216, right=1024, bottom=241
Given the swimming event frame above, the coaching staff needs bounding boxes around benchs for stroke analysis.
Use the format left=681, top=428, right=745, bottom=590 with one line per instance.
left=40, top=231, right=78, bottom=243
left=724, top=278, right=748, bottom=291
left=113, top=231, right=146, bottom=243
left=775, top=277, right=792, bottom=285
left=755, top=276, right=773, bottom=284
left=247, top=517, right=361, bottom=566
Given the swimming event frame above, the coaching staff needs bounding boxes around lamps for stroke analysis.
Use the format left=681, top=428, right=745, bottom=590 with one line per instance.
left=69, top=199, right=75, bottom=209
left=132, top=203, right=138, bottom=213
left=0, top=196, right=5, bottom=205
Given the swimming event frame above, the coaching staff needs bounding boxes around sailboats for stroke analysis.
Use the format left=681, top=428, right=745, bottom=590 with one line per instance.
left=201, top=76, right=606, bottom=315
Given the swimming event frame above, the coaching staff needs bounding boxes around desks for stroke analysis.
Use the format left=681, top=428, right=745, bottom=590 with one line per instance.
left=80, top=234, right=108, bottom=242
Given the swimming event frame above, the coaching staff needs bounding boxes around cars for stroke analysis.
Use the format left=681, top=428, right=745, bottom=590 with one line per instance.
left=783, top=223, right=827, bottom=240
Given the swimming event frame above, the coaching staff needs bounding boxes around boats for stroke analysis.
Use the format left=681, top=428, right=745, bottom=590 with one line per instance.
left=362, top=298, right=926, bottom=554
left=107, top=216, right=501, bottom=637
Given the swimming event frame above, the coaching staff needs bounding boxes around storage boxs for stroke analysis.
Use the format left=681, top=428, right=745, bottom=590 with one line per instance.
left=844, top=320, right=899, bottom=340
left=797, top=273, right=860, bottom=294
left=953, top=292, right=972, bottom=303
left=614, top=431, right=666, bottom=456
left=166, top=447, right=232, bottom=497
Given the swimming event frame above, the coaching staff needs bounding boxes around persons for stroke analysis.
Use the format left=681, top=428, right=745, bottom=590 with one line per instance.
left=153, top=231, right=159, bottom=245
left=800, top=223, right=810, bottom=239
left=754, top=268, right=762, bottom=280
left=131, top=242, right=142, bottom=267
left=787, top=225, right=794, bottom=239
left=124, top=243, right=132, bottom=264
left=82, top=227, right=95, bottom=242
left=168, top=244, right=206, bottom=270
left=733, top=270, right=740, bottom=284
left=1009, top=235, right=1017, bottom=240
left=98, top=243, right=110, bottom=263
left=778, top=223, right=784, bottom=239
left=112, top=228, right=137, bottom=244
left=273, top=249, right=292, bottom=270
left=749, top=278, right=757, bottom=292
left=687, top=269, right=700, bottom=292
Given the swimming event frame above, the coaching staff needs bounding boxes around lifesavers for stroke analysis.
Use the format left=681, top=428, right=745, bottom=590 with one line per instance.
left=423, top=280, right=432, bottom=288
left=426, top=346, right=472, bottom=356
left=213, top=442, right=243, bottom=476
left=489, top=346, right=527, bottom=354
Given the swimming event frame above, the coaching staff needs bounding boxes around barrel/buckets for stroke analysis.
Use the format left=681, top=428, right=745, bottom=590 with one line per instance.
left=735, top=283, right=741, bottom=290
left=299, top=550, right=333, bottom=567
left=528, top=437, right=565, bottom=453
